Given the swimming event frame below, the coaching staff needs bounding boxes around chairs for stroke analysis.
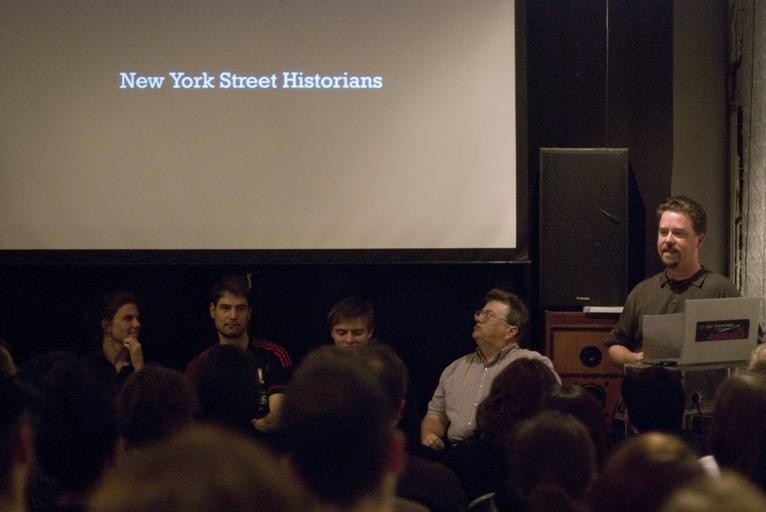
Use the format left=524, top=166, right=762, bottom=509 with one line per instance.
left=469, top=489, right=497, bottom=510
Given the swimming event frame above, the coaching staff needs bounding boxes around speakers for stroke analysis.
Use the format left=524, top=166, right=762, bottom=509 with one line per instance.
left=538, top=147, right=628, bottom=309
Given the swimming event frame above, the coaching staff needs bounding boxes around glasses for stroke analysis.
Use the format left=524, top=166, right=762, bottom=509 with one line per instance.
left=473, top=310, right=516, bottom=323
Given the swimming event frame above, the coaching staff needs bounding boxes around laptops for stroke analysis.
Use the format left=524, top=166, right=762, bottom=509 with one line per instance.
left=642, top=296, right=760, bottom=367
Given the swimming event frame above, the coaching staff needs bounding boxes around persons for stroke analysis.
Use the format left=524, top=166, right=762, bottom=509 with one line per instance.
left=603, top=195, right=740, bottom=364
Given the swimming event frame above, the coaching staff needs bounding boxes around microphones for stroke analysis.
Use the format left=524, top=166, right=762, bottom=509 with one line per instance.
left=597, top=206, right=622, bottom=226
